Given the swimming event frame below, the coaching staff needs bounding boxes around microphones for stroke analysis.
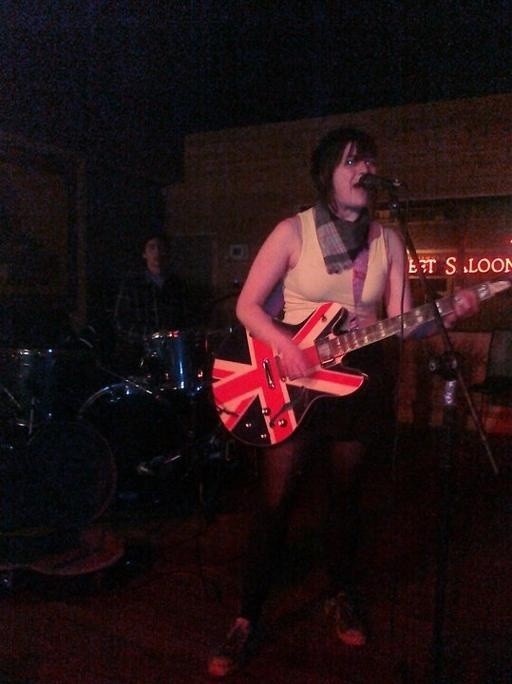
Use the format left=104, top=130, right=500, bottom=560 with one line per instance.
left=359, top=174, right=400, bottom=191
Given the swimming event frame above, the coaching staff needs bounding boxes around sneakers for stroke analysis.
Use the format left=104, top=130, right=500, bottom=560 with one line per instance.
left=207, top=615, right=252, bottom=677
left=310, top=591, right=368, bottom=647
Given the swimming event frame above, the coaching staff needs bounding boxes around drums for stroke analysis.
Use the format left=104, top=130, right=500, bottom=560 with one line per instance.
left=1, top=349, right=92, bottom=429
left=18, top=423, right=117, bottom=524
left=141, top=330, right=232, bottom=394
left=81, top=382, right=190, bottom=509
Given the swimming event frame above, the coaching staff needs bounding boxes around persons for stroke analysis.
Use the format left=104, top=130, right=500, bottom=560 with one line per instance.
left=110, top=234, right=196, bottom=457
left=206, top=125, right=480, bottom=678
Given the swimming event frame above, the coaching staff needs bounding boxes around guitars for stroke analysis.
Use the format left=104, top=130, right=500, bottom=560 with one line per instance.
left=211, top=281, right=511, bottom=446
